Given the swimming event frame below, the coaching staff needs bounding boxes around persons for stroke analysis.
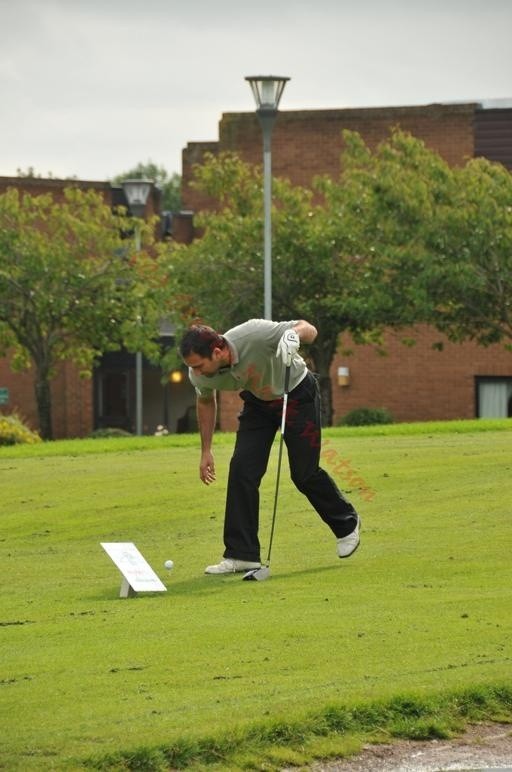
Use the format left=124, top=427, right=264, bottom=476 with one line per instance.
left=180, top=319, right=362, bottom=575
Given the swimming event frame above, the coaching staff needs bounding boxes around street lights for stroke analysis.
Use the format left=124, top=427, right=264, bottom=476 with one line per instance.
left=118, top=179, right=155, bottom=438
left=245, top=75, right=290, bottom=321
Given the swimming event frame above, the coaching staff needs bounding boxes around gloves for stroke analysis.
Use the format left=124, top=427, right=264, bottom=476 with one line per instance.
left=275, top=328, right=299, bottom=364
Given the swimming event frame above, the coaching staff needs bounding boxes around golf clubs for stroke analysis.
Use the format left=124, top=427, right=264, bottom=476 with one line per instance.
left=241, top=367, right=289, bottom=581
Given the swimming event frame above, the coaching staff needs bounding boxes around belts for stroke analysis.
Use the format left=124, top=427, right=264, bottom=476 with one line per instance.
left=287, top=367, right=308, bottom=394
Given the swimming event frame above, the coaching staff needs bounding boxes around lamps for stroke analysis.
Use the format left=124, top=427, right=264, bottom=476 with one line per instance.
left=335, top=364, right=351, bottom=390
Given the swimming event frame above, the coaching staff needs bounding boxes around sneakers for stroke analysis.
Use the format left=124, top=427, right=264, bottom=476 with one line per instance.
left=204, top=558, right=260, bottom=574
left=337, top=516, right=361, bottom=559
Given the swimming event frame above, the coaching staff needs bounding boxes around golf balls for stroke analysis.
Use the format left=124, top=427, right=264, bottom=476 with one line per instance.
left=164, top=560, right=174, bottom=570
